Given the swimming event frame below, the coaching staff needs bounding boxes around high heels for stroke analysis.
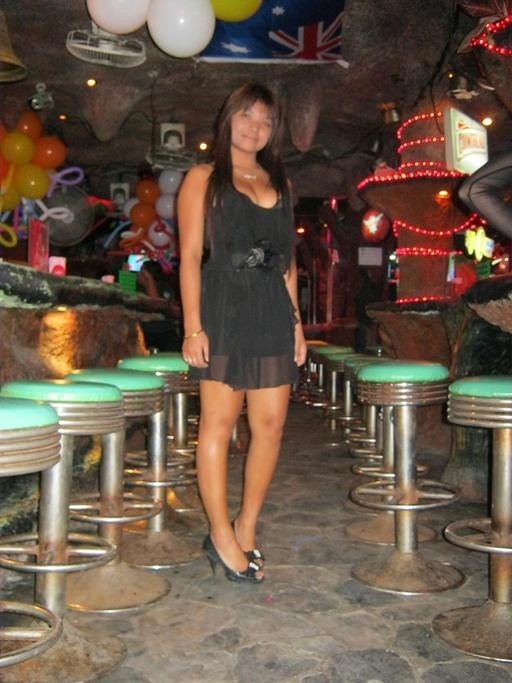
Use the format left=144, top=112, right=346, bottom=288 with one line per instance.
left=229, top=520, right=265, bottom=566
left=201, top=537, right=264, bottom=585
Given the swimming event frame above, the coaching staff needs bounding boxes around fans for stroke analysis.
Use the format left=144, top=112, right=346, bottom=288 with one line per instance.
left=36, top=184, right=95, bottom=247
left=65, top=21, right=150, bottom=68
left=145, top=122, right=198, bottom=172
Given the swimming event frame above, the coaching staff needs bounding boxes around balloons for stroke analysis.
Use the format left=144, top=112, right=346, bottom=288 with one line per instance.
left=103, top=168, right=184, bottom=256
left=84, top=0, right=148, bottom=34
left=147, top=0, right=216, bottom=58
left=211, top=0, right=262, bottom=23
left=1, top=114, right=85, bottom=249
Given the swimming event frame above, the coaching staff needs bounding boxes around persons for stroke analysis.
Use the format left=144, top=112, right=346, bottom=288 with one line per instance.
left=457, top=152, right=512, bottom=239
left=177, top=80, right=308, bottom=585
left=137, top=257, right=164, bottom=298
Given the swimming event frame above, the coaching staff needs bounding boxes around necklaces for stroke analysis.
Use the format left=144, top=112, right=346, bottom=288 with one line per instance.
left=236, top=165, right=261, bottom=180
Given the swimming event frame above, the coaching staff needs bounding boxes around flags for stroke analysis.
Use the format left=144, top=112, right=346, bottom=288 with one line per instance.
left=199, top=0, right=344, bottom=64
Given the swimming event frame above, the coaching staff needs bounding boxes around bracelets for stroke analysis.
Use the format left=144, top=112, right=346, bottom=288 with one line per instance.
left=183, top=328, right=204, bottom=340
